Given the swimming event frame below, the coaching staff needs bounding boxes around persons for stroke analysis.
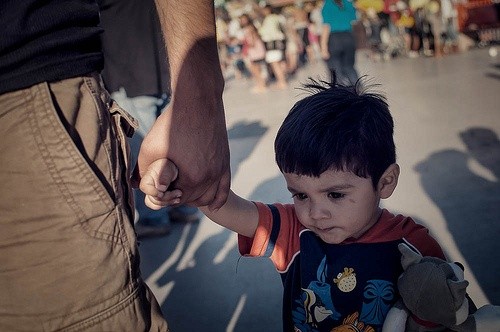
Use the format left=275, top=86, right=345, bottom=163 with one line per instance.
left=216, top=0, right=459, bottom=72
left=261, top=4, right=290, bottom=91
left=140, top=68, right=477, bottom=332
left=320, top=0, right=360, bottom=87
left=99, top=0, right=200, bottom=240
left=0, top=0, right=232, bottom=332
left=238, top=13, right=267, bottom=92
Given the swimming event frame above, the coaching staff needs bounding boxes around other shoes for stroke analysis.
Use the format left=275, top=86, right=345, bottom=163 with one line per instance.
left=134, top=223, right=171, bottom=237
left=167, top=211, right=199, bottom=224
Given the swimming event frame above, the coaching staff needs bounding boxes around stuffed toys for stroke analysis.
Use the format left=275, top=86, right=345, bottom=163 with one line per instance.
left=382, top=243, right=500, bottom=332
